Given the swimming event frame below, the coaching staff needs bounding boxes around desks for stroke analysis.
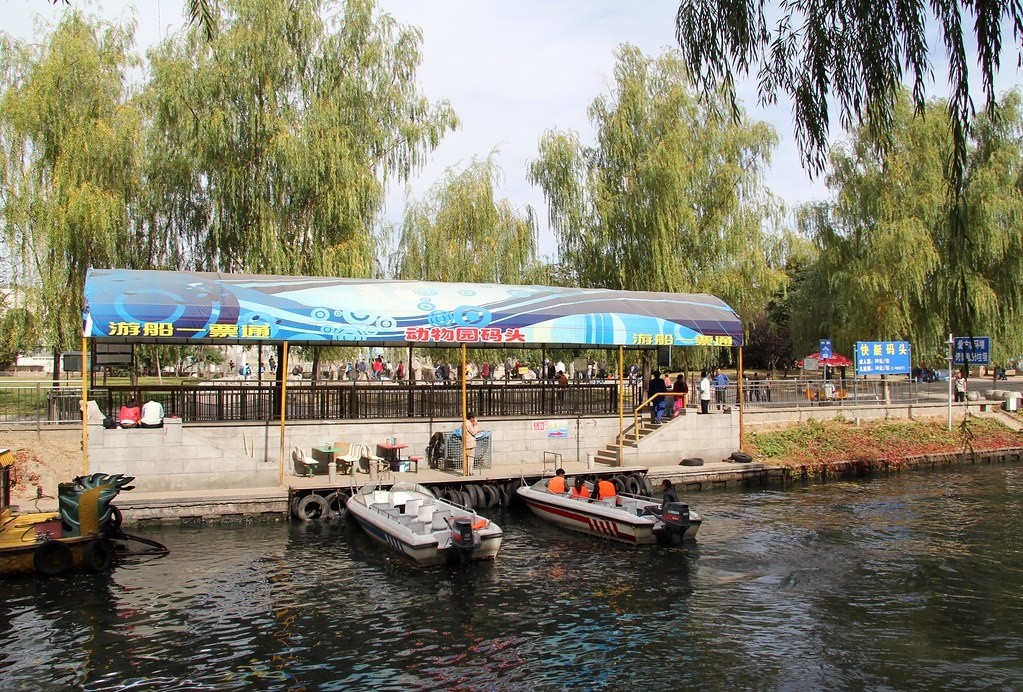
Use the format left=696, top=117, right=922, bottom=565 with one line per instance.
left=312, top=445, right=342, bottom=465
left=377, top=443, right=409, bottom=461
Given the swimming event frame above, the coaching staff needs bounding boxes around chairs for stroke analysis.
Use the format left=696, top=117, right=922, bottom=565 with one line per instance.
left=574, top=495, right=658, bottom=516
left=370, top=499, right=451, bottom=535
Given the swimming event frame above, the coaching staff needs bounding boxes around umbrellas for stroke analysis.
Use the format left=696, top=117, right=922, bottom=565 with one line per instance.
left=796, top=351, right=853, bottom=382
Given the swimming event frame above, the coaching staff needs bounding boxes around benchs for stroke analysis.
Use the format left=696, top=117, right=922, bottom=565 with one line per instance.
left=294, top=445, right=319, bottom=477
left=803, top=387, right=848, bottom=407
left=337, top=442, right=383, bottom=473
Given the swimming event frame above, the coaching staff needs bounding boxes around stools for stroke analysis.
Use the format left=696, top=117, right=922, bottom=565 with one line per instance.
left=409, top=455, right=423, bottom=473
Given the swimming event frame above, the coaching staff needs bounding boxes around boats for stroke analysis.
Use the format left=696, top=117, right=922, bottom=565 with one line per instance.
left=346, top=479, right=503, bottom=568
left=0, top=450, right=135, bottom=572
left=517, top=474, right=703, bottom=549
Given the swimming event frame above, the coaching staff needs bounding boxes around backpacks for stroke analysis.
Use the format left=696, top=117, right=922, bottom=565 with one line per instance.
left=104, top=415, right=117, bottom=429
left=293, top=369, right=297, bottom=375
left=246, top=367, right=251, bottom=375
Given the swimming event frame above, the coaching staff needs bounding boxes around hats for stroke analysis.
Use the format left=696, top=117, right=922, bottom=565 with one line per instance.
left=557, top=370, right=563, bottom=373
left=654, top=370, right=661, bottom=377
left=665, top=373, right=668, bottom=377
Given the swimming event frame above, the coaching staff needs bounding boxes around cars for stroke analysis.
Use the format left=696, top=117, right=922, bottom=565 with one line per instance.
left=907, top=369, right=936, bottom=383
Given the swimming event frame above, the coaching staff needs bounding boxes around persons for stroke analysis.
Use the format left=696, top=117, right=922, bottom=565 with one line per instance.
left=997, top=368, right=1008, bottom=379
left=546, top=469, right=569, bottom=495
left=461, top=412, right=478, bottom=476
left=565, top=476, right=590, bottom=502
left=953, top=372, right=966, bottom=402
left=805, top=367, right=836, bottom=398
left=79, top=399, right=163, bottom=429
left=588, top=475, right=619, bottom=505
left=229, top=357, right=772, bottom=423
left=641, top=479, right=680, bottom=515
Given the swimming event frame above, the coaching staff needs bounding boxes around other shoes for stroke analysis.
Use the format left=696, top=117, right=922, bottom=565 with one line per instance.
left=654, top=418, right=659, bottom=424
